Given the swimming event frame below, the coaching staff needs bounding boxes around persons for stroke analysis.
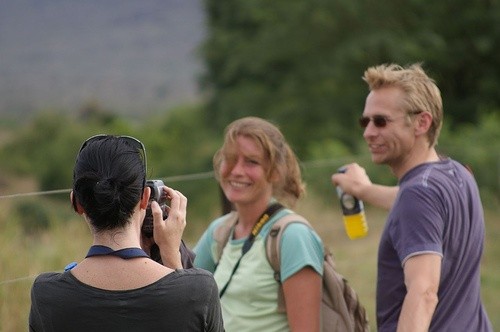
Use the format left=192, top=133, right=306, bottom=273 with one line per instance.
left=331, top=64, right=493, bottom=332
left=28, top=134, right=225, bottom=332
left=192, top=116, right=324, bottom=332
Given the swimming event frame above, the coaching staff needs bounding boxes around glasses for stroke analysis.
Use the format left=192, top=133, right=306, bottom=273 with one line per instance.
left=359, top=111, right=423, bottom=128
left=74, top=135, right=148, bottom=211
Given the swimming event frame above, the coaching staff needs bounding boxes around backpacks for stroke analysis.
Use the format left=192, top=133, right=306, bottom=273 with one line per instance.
left=214, top=209, right=370, bottom=332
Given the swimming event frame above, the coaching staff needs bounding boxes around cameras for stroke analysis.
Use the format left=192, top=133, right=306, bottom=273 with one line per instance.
left=144, top=179, right=166, bottom=210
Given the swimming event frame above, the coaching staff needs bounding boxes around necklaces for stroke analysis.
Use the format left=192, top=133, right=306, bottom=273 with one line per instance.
left=212, top=202, right=287, bottom=298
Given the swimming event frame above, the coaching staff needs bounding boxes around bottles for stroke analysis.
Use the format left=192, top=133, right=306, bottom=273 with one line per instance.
left=336, top=166, right=368, bottom=239
